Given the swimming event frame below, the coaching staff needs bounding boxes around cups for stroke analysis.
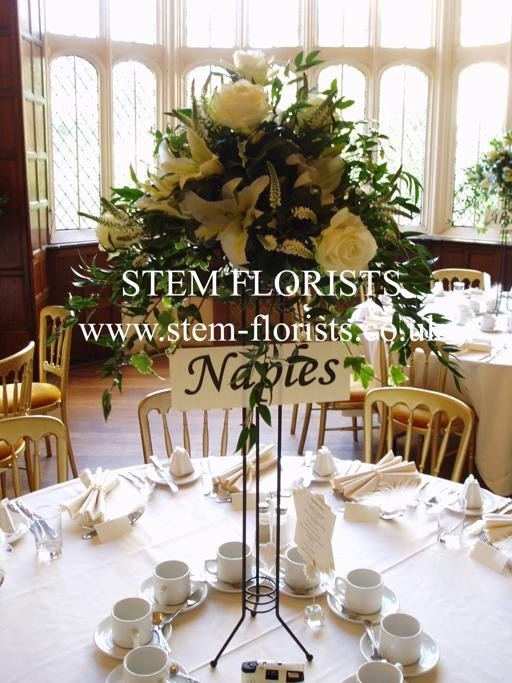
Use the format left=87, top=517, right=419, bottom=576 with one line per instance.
left=434, top=281, right=512, bottom=332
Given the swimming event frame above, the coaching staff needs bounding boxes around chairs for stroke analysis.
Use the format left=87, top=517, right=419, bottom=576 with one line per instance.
left=0, top=303, right=79, bottom=502
left=349, top=291, right=512, bottom=495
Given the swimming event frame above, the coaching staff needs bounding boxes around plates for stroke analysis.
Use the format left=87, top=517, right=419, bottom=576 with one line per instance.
left=1, top=457, right=512, bottom=681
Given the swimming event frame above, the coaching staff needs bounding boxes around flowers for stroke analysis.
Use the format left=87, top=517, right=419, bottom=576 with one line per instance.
left=47, top=45, right=481, bottom=455
left=445, top=130, right=512, bottom=234
